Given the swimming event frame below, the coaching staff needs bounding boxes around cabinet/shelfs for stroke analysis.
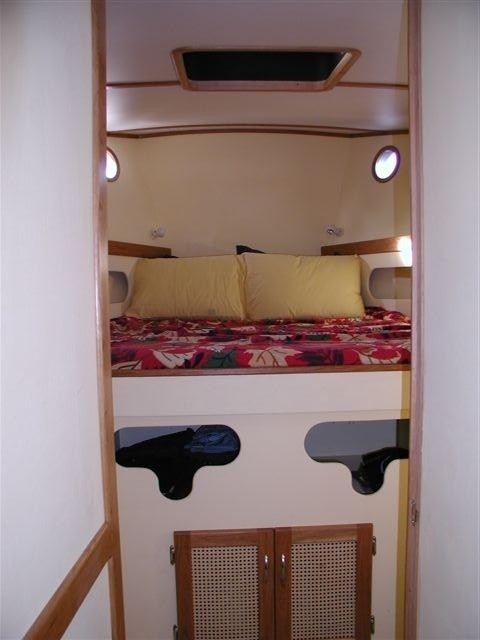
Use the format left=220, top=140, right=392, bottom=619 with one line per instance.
left=168, top=523, right=377, bottom=639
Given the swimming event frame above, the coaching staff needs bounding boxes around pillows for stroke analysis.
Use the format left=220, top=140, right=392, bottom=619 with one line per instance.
left=124, top=252, right=366, bottom=321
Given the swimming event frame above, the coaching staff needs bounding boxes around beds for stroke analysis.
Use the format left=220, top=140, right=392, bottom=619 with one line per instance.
left=110, top=236, right=411, bottom=371
